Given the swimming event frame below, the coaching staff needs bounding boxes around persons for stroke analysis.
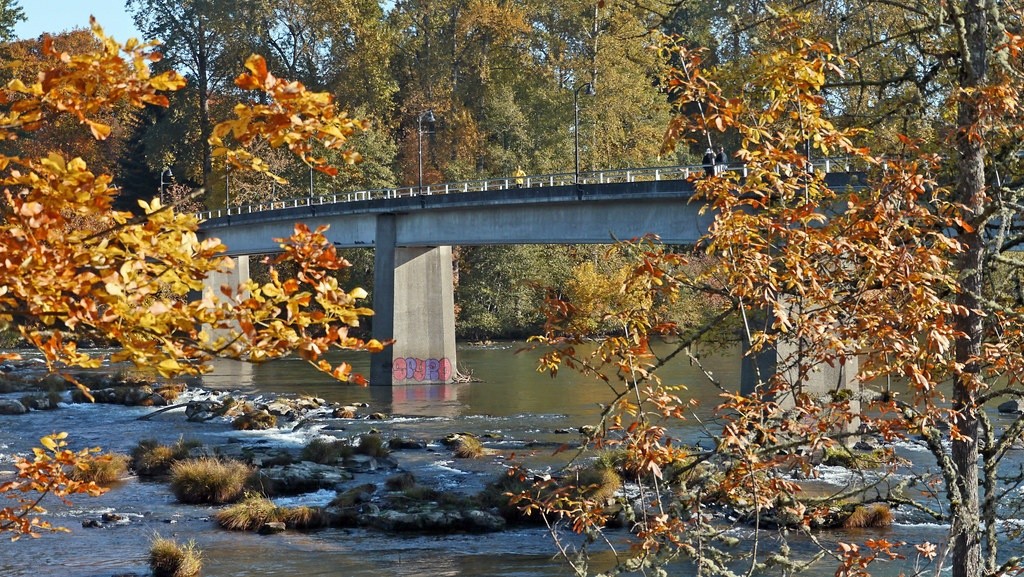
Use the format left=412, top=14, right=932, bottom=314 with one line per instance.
left=714, top=145, right=729, bottom=179
left=702, top=147, right=716, bottom=178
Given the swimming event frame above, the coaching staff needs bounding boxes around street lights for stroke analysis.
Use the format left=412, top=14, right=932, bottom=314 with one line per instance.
left=159, top=168, right=174, bottom=205
left=419, top=109, right=435, bottom=194
left=225, top=149, right=241, bottom=217
left=574, top=82, right=596, bottom=183
